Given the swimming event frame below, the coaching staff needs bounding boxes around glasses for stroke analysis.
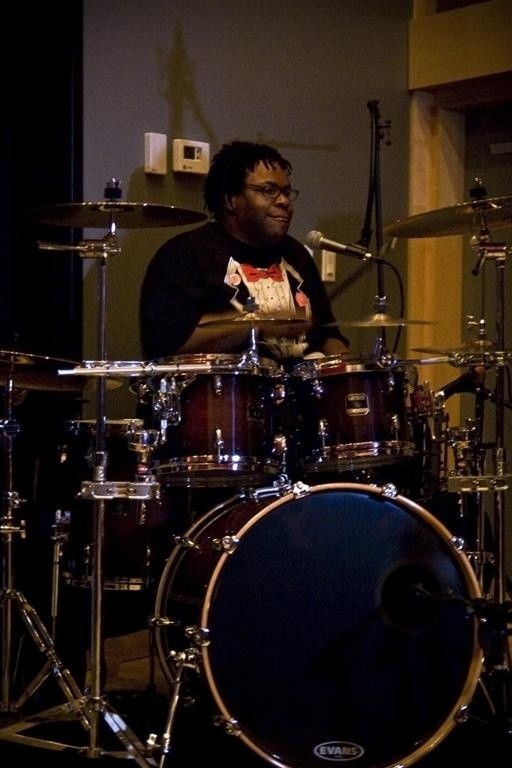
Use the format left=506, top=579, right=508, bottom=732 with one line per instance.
left=247, top=183, right=299, bottom=201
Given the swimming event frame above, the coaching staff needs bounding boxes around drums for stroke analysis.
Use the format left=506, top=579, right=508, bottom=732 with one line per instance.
left=69, top=482, right=164, bottom=592
left=289, top=352, right=415, bottom=472
left=129, top=355, right=286, bottom=488
left=153, top=481, right=487, bottom=765
left=60, top=418, right=151, bottom=481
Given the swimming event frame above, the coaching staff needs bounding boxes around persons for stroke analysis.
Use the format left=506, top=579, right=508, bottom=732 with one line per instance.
left=138, top=138, right=352, bottom=359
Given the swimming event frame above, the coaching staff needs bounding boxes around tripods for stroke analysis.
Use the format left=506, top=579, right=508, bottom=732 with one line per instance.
left=0, top=257, right=162, bottom=768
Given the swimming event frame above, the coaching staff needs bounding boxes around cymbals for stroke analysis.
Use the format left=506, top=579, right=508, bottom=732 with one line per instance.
left=324, top=314, right=441, bottom=326
left=0, top=352, right=124, bottom=392
left=415, top=342, right=510, bottom=356
left=384, top=198, right=512, bottom=237
left=197, top=313, right=308, bottom=327
left=31, top=202, right=208, bottom=228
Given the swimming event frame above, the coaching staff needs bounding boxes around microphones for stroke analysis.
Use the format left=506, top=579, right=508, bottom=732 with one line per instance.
left=433, top=356, right=496, bottom=406
left=305, top=230, right=385, bottom=264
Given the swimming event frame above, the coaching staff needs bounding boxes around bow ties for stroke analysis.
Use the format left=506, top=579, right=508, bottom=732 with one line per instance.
left=241, top=263, right=284, bottom=282
